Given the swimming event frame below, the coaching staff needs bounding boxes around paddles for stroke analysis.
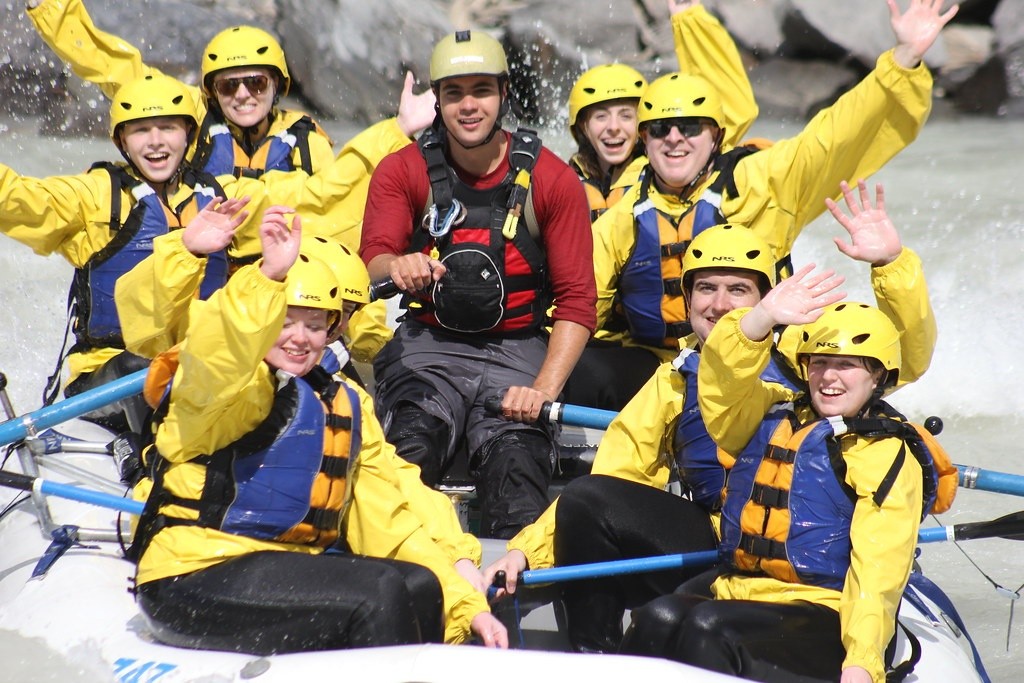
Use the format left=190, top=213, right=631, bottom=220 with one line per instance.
left=0, top=257, right=454, bottom=518
left=485, top=393, right=1024, bottom=587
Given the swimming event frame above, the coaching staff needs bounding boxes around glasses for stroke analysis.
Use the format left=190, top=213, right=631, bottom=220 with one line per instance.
left=643, top=116, right=717, bottom=138
left=212, top=74, right=275, bottom=97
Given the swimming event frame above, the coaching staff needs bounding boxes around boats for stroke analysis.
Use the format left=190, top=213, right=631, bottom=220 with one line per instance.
left=0, top=395, right=985, bottom=683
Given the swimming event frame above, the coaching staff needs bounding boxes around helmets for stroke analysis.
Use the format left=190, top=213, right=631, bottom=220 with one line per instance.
left=109, top=75, right=202, bottom=147
left=200, top=26, right=290, bottom=99
left=636, top=72, right=726, bottom=140
left=429, top=30, right=509, bottom=81
left=796, top=303, right=902, bottom=389
left=680, top=223, right=776, bottom=302
left=301, top=234, right=375, bottom=303
left=286, top=253, right=342, bottom=339
left=566, top=62, right=646, bottom=126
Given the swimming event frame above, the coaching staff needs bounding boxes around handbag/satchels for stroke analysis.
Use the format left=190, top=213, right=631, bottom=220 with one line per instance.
left=435, top=243, right=506, bottom=333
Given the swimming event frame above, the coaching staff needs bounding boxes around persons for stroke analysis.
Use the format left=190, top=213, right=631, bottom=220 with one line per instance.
left=359, top=31, right=597, bottom=539
left=483, top=182, right=938, bottom=668
left=131, top=203, right=511, bottom=657
left=630, top=262, right=923, bottom=682
left=0, top=0, right=491, bottom=595
left=556, top=0, right=961, bottom=411
